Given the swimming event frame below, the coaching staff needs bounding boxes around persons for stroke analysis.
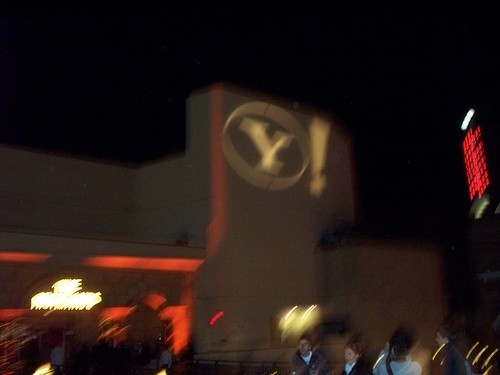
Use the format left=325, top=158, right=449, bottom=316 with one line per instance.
left=341, top=341, right=374, bottom=374
left=429, top=328, right=472, bottom=374
left=371, top=329, right=423, bottom=374
left=290, top=337, right=330, bottom=374
left=51, top=336, right=191, bottom=375
